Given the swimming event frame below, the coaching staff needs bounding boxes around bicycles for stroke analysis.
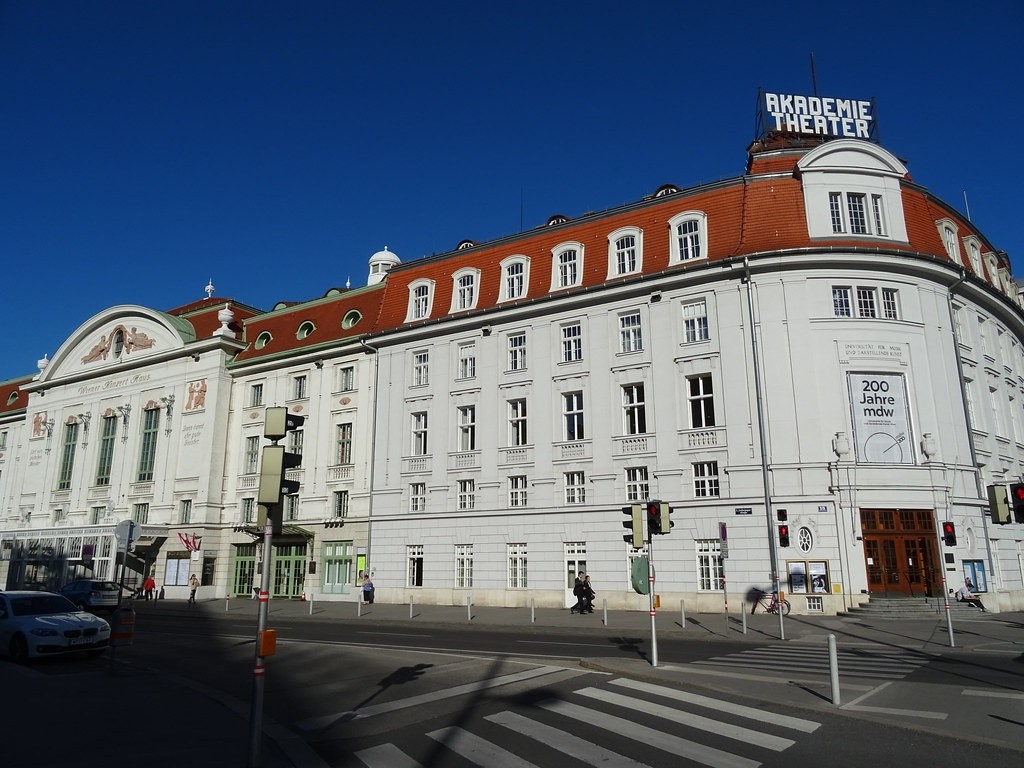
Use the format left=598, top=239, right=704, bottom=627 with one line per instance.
left=751, top=587, right=791, bottom=616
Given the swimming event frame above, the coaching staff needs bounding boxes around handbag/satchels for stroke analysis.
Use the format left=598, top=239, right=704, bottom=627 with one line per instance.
left=194, top=579, right=200, bottom=587
left=369, top=582, right=373, bottom=588
left=573, top=587, right=577, bottom=596
left=360, top=586, right=364, bottom=592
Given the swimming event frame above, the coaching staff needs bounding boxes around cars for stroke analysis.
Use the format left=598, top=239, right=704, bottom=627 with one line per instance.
left=62, top=582, right=138, bottom=610
left=0, top=590, right=110, bottom=663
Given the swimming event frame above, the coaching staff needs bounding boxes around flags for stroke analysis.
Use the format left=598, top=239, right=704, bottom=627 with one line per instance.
left=178, top=532, right=198, bottom=553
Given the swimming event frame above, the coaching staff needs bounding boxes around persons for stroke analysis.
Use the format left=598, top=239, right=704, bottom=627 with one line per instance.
left=188, top=574, right=199, bottom=604
left=125, top=326, right=137, bottom=340
left=98, top=335, right=109, bottom=350
left=362, top=575, right=372, bottom=605
left=957, top=583, right=991, bottom=612
left=570, top=571, right=596, bottom=614
left=143, top=576, right=155, bottom=601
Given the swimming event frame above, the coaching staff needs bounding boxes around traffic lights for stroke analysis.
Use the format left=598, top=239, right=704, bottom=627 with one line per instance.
left=622, top=504, right=643, bottom=548
left=1009, top=483, right=1024, bottom=525
left=778, top=525, right=789, bottom=547
left=646, top=502, right=674, bottom=533
left=259, top=407, right=305, bottom=504
left=942, top=522, right=957, bottom=546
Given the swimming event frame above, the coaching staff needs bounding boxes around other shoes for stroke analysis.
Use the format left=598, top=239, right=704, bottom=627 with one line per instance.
left=588, top=608, right=594, bottom=613
left=580, top=612, right=587, bottom=615
left=571, top=607, right=574, bottom=614
